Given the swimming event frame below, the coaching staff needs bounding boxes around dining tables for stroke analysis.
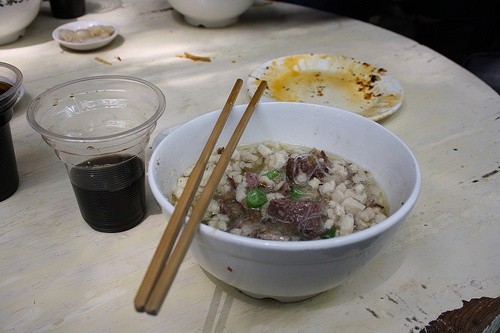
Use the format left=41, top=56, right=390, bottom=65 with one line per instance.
left=0, top=0, right=500, bottom=333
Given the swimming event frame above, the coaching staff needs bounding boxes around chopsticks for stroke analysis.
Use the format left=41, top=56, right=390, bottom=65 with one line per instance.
left=133, top=79, right=267, bottom=316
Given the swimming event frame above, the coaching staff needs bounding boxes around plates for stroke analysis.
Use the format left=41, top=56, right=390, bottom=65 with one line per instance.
left=246, top=53, right=404, bottom=123
left=52, top=20, right=119, bottom=51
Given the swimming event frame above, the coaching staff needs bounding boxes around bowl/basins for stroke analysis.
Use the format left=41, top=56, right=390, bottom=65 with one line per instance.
left=0, top=0, right=41, bottom=47
left=168, top=0, right=255, bottom=29
left=148, top=102, right=423, bottom=304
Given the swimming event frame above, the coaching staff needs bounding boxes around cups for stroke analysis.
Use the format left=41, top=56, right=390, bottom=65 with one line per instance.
left=26, top=75, right=166, bottom=233
left=48, top=0, right=86, bottom=20
left=0, top=61, right=24, bottom=203
